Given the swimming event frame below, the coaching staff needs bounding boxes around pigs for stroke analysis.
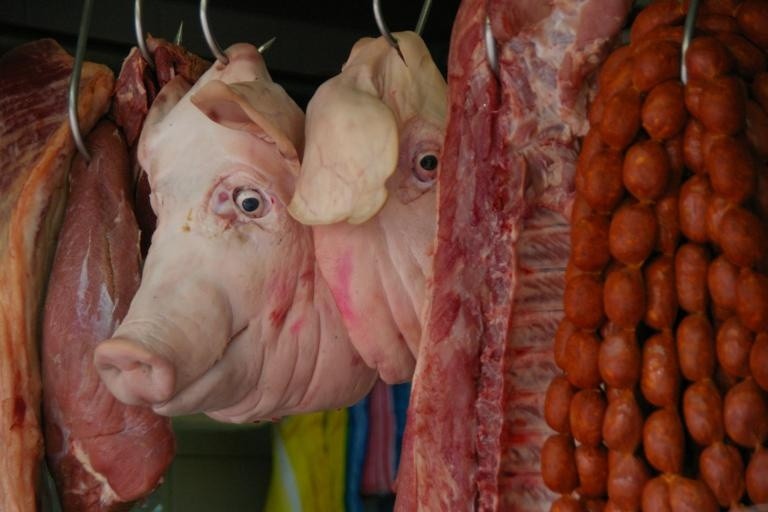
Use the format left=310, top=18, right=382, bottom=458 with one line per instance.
left=284, top=29, right=447, bottom=388
left=91, top=41, right=378, bottom=427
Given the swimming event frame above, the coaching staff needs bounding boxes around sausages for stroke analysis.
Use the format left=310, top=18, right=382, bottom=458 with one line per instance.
left=539, top=0, right=768, bottom=512
